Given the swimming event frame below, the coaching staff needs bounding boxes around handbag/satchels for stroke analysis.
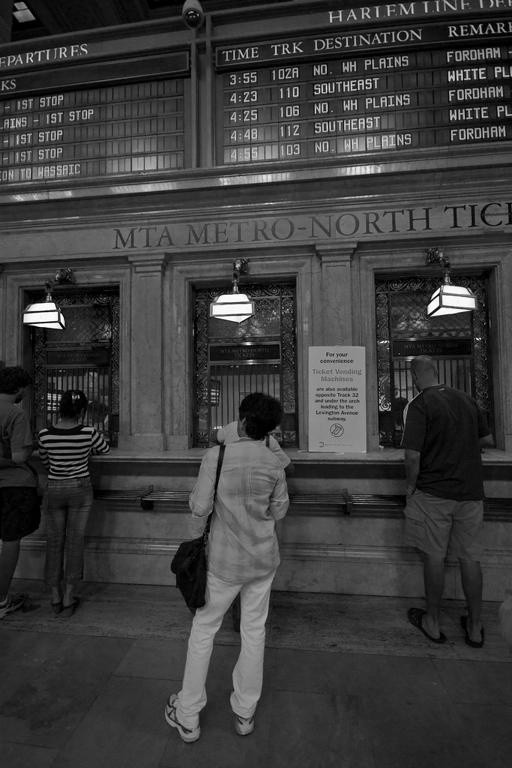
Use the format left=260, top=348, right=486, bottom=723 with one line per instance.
left=170, top=537, right=207, bottom=615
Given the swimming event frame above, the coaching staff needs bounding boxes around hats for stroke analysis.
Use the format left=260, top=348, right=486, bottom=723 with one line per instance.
left=60, top=389, right=94, bottom=409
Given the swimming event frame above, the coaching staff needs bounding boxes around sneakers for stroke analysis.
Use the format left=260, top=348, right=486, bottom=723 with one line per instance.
left=1, top=593, right=28, bottom=620
left=227, top=688, right=256, bottom=736
left=162, top=693, right=203, bottom=743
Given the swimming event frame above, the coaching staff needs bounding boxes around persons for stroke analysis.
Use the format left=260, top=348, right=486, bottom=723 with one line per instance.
left=381, top=396, right=409, bottom=447
left=212, top=419, right=292, bottom=471
left=36, top=390, right=110, bottom=618
left=402, top=356, right=494, bottom=648
left=164, top=393, right=291, bottom=740
left=0, top=366, right=42, bottom=618
left=84, top=400, right=109, bottom=440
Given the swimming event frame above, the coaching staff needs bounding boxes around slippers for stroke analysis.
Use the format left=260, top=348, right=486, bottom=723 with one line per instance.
left=50, top=599, right=62, bottom=615
left=62, top=596, right=80, bottom=617
left=407, top=606, right=447, bottom=644
left=459, top=615, right=486, bottom=649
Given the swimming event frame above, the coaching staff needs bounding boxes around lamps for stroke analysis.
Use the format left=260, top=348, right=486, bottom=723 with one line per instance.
left=207, top=257, right=261, bottom=323
left=418, top=244, right=481, bottom=318
left=19, top=264, right=80, bottom=334
